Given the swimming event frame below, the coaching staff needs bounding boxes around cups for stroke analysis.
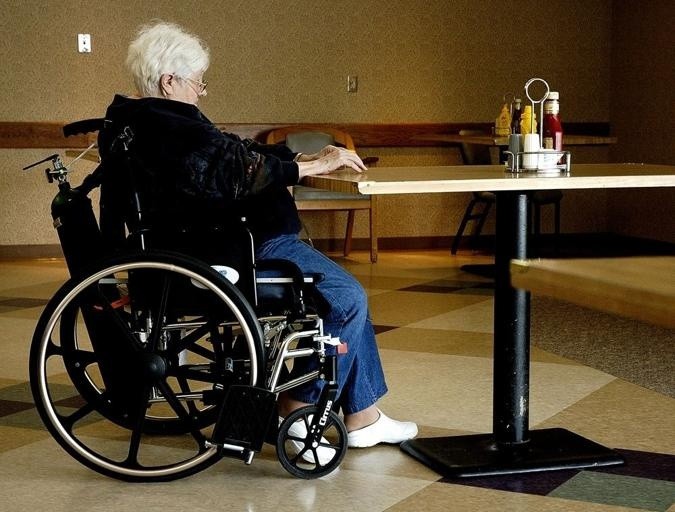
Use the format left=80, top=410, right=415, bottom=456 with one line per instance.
left=538, top=149, right=562, bottom=176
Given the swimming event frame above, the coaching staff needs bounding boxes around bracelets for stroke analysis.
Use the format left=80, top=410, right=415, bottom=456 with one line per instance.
left=292, top=150, right=305, bottom=163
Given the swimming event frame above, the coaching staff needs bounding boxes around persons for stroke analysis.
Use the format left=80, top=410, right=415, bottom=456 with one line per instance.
left=95, top=14, right=420, bottom=466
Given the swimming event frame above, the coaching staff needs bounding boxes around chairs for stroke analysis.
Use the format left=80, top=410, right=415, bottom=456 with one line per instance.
left=450, top=129, right=564, bottom=254
left=263, top=126, right=377, bottom=262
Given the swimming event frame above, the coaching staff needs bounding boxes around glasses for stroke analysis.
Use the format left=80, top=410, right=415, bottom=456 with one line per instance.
left=173, top=72, right=209, bottom=93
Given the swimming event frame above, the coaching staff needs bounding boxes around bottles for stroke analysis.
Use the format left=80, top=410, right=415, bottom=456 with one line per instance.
left=496, top=103, right=511, bottom=136
left=541, top=91, right=564, bottom=150
left=513, top=99, right=523, bottom=133
left=508, top=133, right=523, bottom=168
left=523, top=132, right=540, bottom=171
left=520, top=105, right=538, bottom=146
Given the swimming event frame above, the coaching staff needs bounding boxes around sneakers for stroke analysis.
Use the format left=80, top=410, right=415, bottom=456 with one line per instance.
left=277, top=413, right=337, bottom=466
left=347, top=407, right=418, bottom=449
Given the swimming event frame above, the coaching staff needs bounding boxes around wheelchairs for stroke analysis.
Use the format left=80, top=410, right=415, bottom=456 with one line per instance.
left=23, top=117, right=350, bottom=487
